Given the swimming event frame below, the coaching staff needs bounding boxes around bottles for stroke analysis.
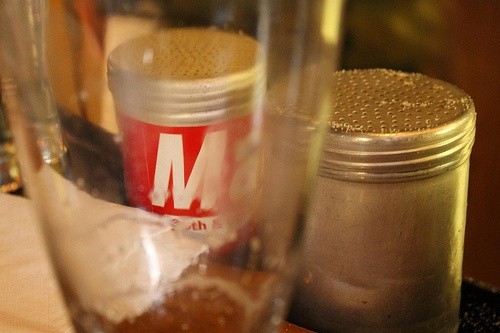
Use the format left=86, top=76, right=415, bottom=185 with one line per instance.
left=267, top=59, right=475, bottom=333
left=107, top=27, right=269, bottom=308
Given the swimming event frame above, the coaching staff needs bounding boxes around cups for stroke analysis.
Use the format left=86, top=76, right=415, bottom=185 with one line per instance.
left=0, top=2, right=338, bottom=333
